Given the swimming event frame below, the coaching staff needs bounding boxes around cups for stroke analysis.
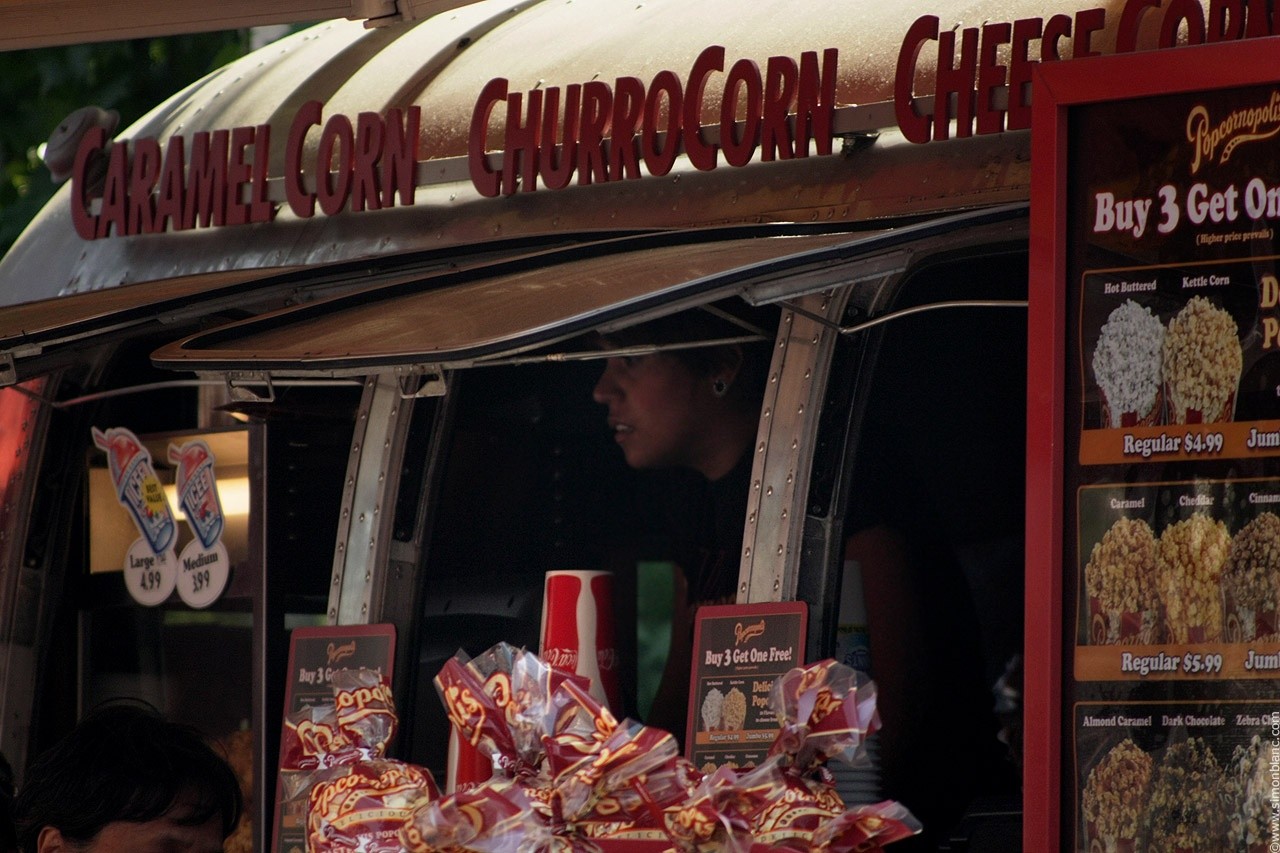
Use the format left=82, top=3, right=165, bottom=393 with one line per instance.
left=538, top=569, right=621, bottom=742
left=447, top=718, right=495, bottom=795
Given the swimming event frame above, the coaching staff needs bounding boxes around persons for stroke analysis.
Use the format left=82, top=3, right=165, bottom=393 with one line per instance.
left=577, top=297, right=914, bottom=768
left=1, top=693, right=243, bottom=853
